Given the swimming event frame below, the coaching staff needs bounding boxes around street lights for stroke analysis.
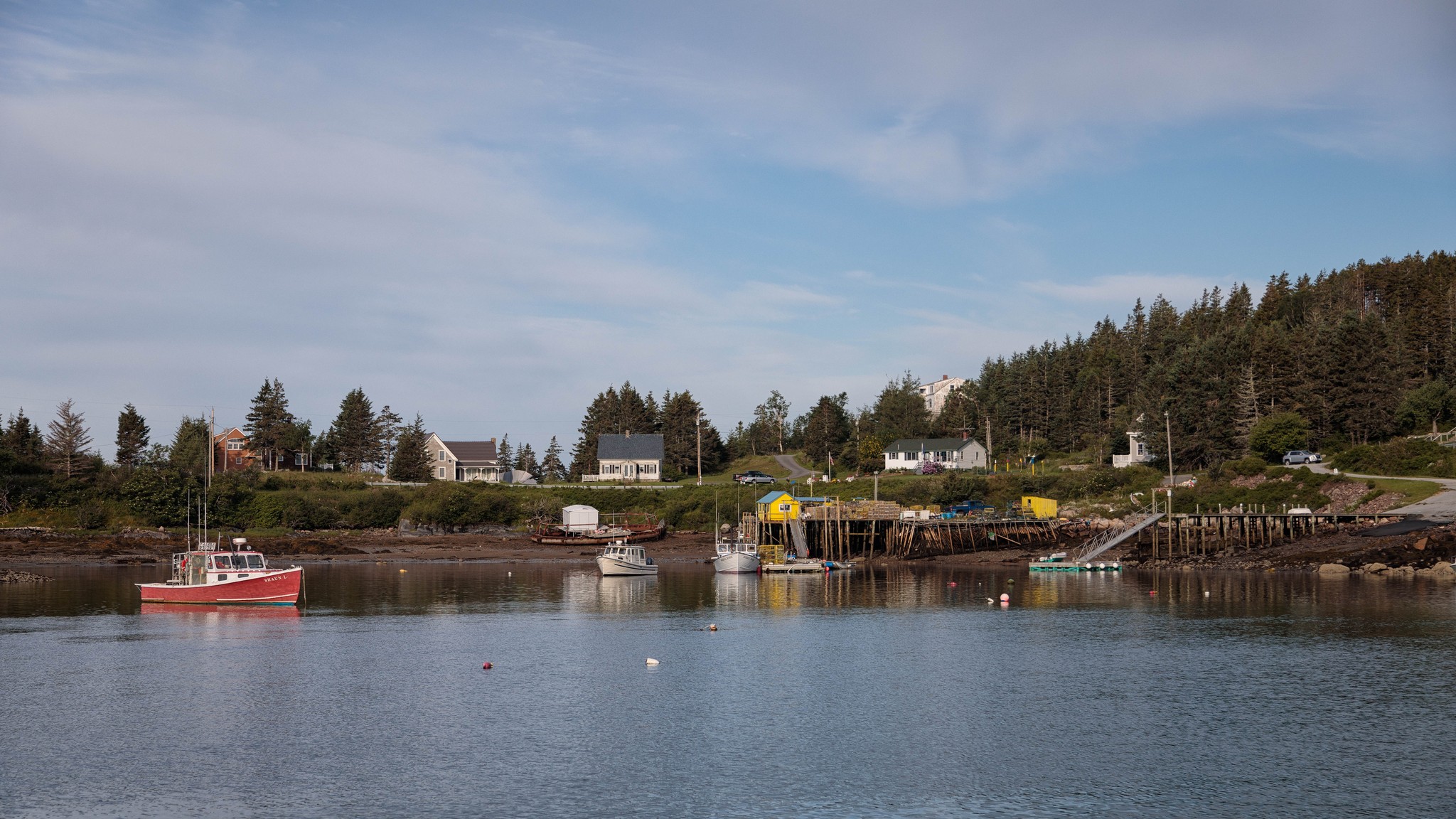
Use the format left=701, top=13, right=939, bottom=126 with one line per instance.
left=1164, top=411, right=1173, bottom=484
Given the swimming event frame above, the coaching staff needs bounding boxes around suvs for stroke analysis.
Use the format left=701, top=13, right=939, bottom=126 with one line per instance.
left=1283, top=450, right=1322, bottom=465
left=1024, top=511, right=1035, bottom=519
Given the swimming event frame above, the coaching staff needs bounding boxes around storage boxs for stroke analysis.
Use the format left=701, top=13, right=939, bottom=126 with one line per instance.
left=757, top=545, right=784, bottom=565
left=805, top=501, right=940, bottom=519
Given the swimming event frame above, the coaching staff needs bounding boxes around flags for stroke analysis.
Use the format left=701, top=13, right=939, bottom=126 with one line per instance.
left=830, top=454, right=834, bottom=466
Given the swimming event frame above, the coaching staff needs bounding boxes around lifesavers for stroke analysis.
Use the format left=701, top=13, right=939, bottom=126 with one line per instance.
left=182, top=559, right=191, bottom=572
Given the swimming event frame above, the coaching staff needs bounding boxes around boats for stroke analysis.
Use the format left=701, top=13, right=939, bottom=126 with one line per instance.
left=819, top=560, right=857, bottom=569
left=710, top=471, right=762, bottom=573
left=595, top=509, right=659, bottom=576
left=134, top=448, right=302, bottom=604
left=1040, top=552, right=1067, bottom=563
left=530, top=505, right=667, bottom=544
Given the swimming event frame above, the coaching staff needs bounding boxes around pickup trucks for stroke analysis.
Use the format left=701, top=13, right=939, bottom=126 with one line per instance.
left=732, top=471, right=773, bottom=484
left=943, top=501, right=995, bottom=516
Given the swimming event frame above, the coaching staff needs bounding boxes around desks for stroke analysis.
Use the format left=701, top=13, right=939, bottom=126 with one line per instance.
left=992, top=513, right=1006, bottom=520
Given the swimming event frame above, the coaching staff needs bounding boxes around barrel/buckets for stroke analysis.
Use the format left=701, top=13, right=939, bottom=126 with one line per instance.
left=940, top=514, right=946, bottom=519
left=951, top=511, right=956, bottom=518
left=946, top=511, right=952, bottom=519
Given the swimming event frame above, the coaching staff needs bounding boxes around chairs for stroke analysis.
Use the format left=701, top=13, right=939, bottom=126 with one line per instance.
left=467, top=475, right=494, bottom=481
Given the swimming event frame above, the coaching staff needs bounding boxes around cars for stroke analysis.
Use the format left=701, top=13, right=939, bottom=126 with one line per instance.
left=741, top=474, right=778, bottom=484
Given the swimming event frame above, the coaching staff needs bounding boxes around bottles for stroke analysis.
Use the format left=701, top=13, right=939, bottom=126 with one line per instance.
left=483, top=662, right=493, bottom=668
